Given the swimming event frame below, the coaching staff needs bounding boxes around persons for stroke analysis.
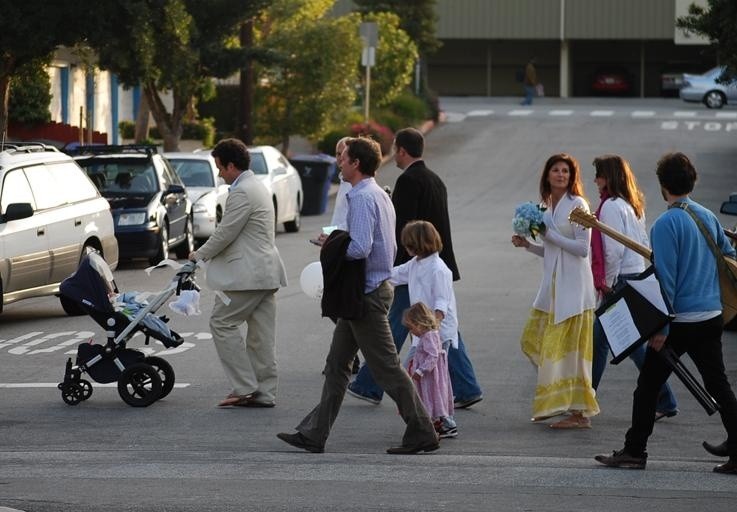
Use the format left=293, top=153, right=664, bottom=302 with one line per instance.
left=277, top=137, right=440, bottom=455
left=188, top=139, right=288, bottom=409
left=595, top=153, right=737, bottom=475
left=511, top=154, right=600, bottom=429
left=322, top=137, right=360, bottom=375
left=392, top=221, right=459, bottom=437
left=402, top=302, right=455, bottom=431
left=589, top=156, right=679, bottom=422
left=347, top=128, right=482, bottom=409
left=520, top=58, right=537, bottom=104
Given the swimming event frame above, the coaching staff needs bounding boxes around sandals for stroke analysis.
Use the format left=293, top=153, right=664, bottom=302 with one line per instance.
left=530, top=413, right=563, bottom=421
left=654, top=409, right=679, bottom=421
left=548, top=414, right=592, bottom=428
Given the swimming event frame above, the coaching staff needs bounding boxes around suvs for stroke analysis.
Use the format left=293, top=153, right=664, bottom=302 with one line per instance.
left=0, top=143, right=120, bottom=315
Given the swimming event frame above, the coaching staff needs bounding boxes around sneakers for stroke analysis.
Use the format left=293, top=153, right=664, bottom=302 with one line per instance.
left=434, top=423, right=457, bottom=438
left=454, top=389, right=482, bottom=408
left=346, top=382, right=381, bottom=405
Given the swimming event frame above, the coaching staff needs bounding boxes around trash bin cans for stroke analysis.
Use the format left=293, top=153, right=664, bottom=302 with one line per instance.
left=289, top=153, right=338, bottom=216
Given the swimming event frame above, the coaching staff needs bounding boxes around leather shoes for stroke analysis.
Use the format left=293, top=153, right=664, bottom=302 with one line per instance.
left=277, top=431, right=324, bottom=453
left=713, top=460, right=737, bottom=475
left=386, top=440, right=439, bottom=454
left=703, top=441, right=729, bottom=457
left=595, top=448, right=648, bottom=469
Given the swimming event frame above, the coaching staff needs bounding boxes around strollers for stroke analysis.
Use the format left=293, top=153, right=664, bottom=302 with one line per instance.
left=55, top=251, right=205, bottom=405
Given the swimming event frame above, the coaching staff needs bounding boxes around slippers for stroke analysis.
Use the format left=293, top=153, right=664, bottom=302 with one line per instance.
left=217, top=392, right=256, bottom=406
left=232, top=399, right=275, bottom=407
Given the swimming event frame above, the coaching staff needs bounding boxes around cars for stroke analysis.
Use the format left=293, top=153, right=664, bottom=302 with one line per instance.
left=72, top=147, right=193, bottom=265
left=661, top=64, right=684, bottom=90
left=679, top=65, right=737, bottom=109
left=245, top=146, right=305, bottom=232
left=592, top=64, right=630, bottom=92
left=162, top=152, right=231, bottom=239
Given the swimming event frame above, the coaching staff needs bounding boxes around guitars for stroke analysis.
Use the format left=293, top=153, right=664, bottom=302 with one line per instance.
left=566, top=205, right=737, bottom=325
